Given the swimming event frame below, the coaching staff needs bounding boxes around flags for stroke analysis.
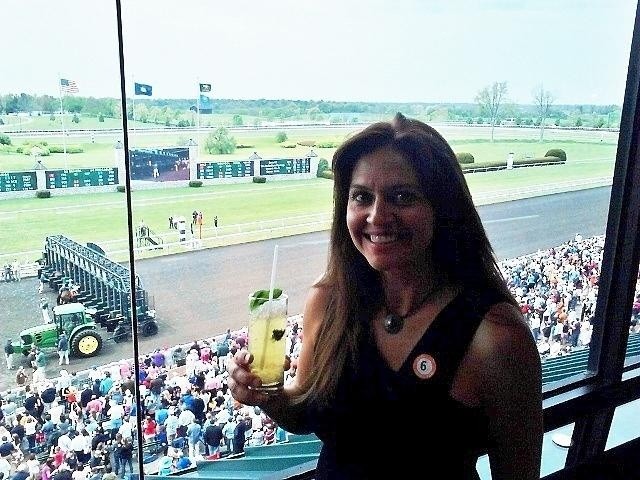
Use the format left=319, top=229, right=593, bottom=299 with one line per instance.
left=61, top=80, right=79, bottom=94
left=201, top=83, right=210, bottom=93
left=134, top=82, right=152, bottom=95
left=201, top=95, right=209, bottom=102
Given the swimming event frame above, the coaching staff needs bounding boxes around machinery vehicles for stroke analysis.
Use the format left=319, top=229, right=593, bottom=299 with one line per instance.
left=4, top=287, right=103, bottom=368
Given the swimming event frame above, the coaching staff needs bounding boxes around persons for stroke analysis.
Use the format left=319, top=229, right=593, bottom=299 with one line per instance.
left=1, top=358, right=140, bottom=480
left=40, top=296, right=52, bottom=324
left=500, top=231, right=640, bottom=357
left=139, top=220, right=146, bottom=234
left=138, top=313, right=305, bottom=478
left=169, top=210, right=219, bottom=228
left=3, top=258, right=22, bottom=283
left=15, top=344, right=46, bottom=387
left=4, top=340, right=15, bottom=370
left=57, top=333, right=70, bottom=366
left=130, top=147, right=189, bottom=182
left=57, top=280, right=81, bottom=306
left=37, top=266, right=45, bottom=294
left=225, top=113, right=541, bottom=480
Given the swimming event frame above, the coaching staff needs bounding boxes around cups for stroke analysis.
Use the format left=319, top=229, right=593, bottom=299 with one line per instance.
left=243, top=290, right=291, bottom=397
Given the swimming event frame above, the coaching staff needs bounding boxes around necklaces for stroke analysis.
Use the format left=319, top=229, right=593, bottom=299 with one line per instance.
left=377, top=287, right=437, bottom=335
